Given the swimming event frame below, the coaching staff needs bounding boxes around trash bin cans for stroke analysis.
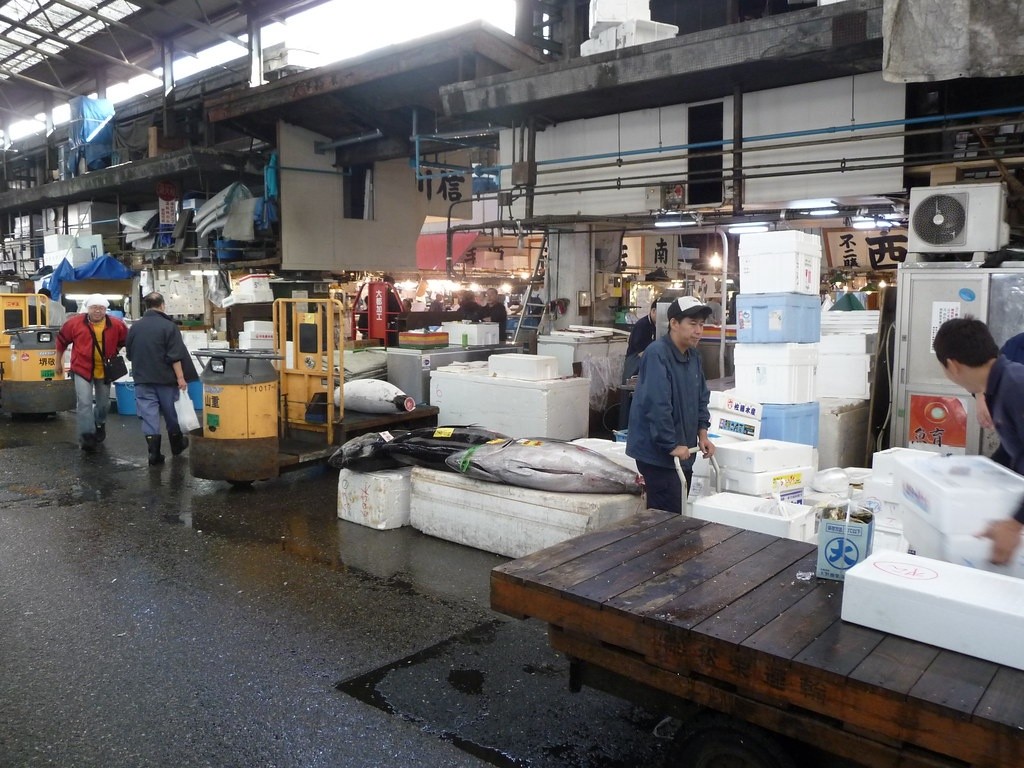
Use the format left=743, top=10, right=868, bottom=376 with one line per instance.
left=113, top=381, right=139, bottom=415
left=187, top=380, right=203, bottom=409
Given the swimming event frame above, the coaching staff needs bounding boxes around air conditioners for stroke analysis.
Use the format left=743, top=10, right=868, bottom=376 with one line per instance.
left=907, top=180, right=1010, bottom=251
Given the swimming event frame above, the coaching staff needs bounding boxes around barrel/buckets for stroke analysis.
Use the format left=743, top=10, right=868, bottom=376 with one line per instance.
left=113, top=381, right=138, bottom=416
left=186, top=376, right=204, bottom=410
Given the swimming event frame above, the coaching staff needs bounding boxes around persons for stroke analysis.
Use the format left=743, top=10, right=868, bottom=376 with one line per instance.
left=55, top=293, right=128, bottom=449
left=358, top=276, right=413, bottom=347
left=619, top=298, right=657, bottom=431
left=126, top=291, right=189, bottom=464
left=625, top=296, right=716, bottom=512
left=934, top=316, right=1024, bottom=566
left=474, top=288, right=507, bottom=344
left=859, top=271, right=878, bottom=291
left=457, top=291, right=484, bottom=322
left=38, top=288, right=66, bottom=327
left=428, top=294, right=442, bottom=325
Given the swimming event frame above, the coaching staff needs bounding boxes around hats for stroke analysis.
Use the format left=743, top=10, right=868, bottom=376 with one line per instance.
left=667, top=295, right=712, bottom=320
left=83, top=294, right=110, bottom=308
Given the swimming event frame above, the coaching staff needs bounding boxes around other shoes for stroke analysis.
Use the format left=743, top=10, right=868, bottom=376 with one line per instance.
left=80, top=423, right=106, bottom=451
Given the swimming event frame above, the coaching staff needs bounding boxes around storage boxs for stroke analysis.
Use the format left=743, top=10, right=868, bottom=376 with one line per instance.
left=336, top=230, right=1024, bottom=671
left=238, top=321, right=276, bottom=350
left=221, top=273, right=274, bottom=311
left=42, top=233, right=103, bottom=271
left=580, top=0, right=678, bottom=57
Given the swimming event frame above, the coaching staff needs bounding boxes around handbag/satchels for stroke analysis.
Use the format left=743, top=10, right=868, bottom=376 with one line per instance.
left=104, top=356, right=128, bottom=385
left=174, top=386, right=200, bottom=433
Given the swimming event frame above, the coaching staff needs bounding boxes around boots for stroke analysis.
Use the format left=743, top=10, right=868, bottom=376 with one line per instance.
left=168, top=424, right=189, bottom=455
left=144, top=434, right=166, bottom=466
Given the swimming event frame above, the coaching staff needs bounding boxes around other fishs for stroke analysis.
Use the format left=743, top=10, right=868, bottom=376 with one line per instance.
left=328, top=378, right=646, bottom=496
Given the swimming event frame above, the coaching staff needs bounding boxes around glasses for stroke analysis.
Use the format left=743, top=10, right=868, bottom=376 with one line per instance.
left=91, top=306, right=106, bottom=312
